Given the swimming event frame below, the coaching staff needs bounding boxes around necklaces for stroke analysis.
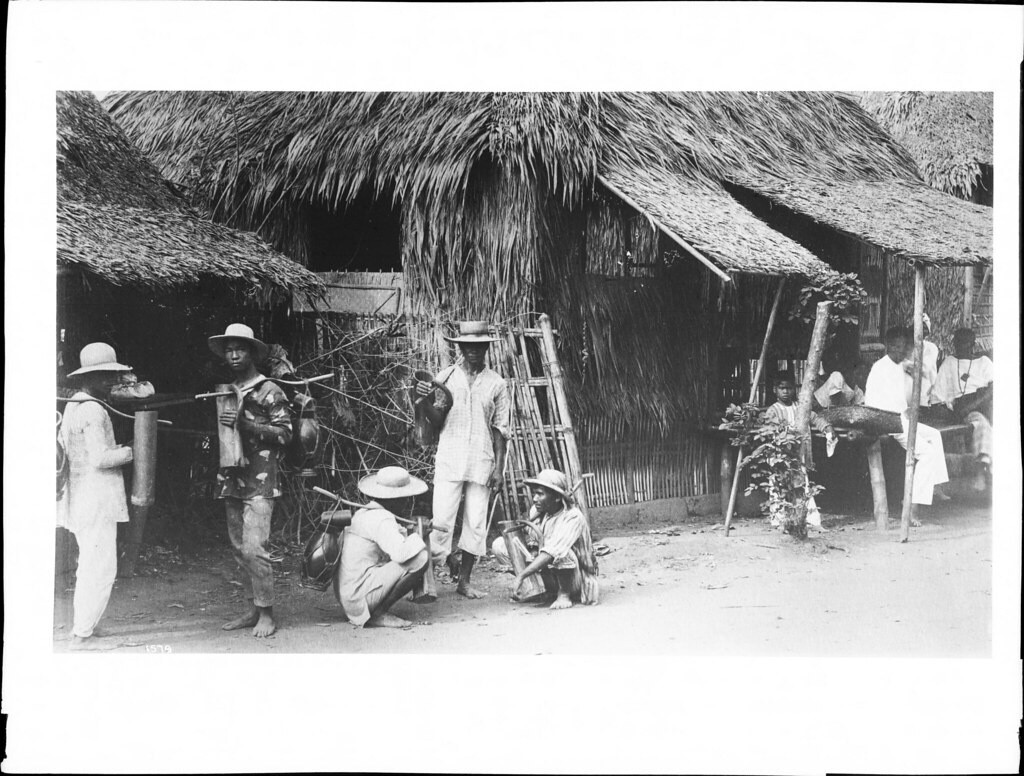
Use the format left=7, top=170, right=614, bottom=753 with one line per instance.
left=956, top=350, right=973, bottom=402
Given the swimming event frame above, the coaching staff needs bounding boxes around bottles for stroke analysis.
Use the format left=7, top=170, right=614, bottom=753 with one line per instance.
left=57, top=412, right=69, bottom=499
left=303, top=523, right=337, bottom=583
left=291, top=410, right=323, bottom=469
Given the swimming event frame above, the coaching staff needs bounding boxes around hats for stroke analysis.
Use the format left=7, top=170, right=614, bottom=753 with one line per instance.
left=208, top=322, right=270, bottom=365
left=523, top=468, right=575, bottom=506
left=443, top=319, right=503, bottom=344
left=66, top=342, right=134, bottom=379
left=358, top=465, right=429, bottom=499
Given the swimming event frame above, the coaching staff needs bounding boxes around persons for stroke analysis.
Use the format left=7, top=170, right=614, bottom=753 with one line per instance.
left=406, top=321, right=513, bottom=602
left=492, top=469, right=601, bottom=610
left=810, top=344, right=872, bottom=414
left=207, top=322, right=294, bottom=637
left=931, top=327, right=993, bottom=484
left=908, top=321, right=939, bottom=387
left=863, top=326, right=950, bottom=527
left=56, top=342, right=136, bottom=650
left=763, top=370, right=837, bottom=531
left=333, top=466, right=433, bottom=627
